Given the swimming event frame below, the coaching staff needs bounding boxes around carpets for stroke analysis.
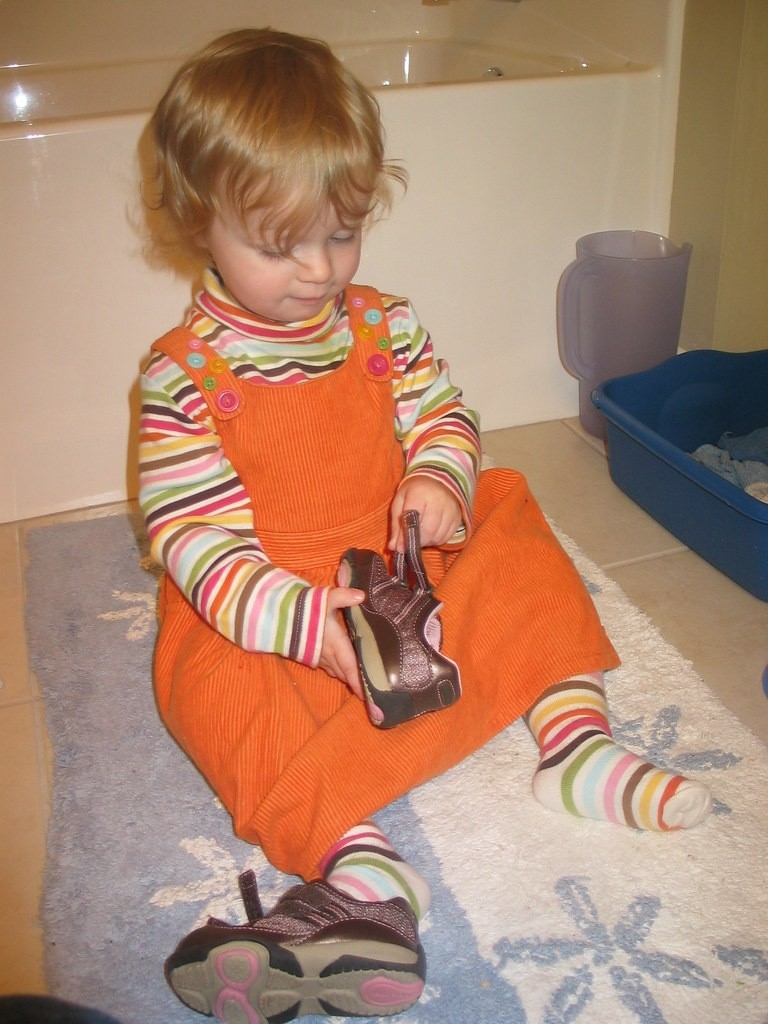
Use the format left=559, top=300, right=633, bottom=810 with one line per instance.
left=26, top=505, right=768, bottom=1024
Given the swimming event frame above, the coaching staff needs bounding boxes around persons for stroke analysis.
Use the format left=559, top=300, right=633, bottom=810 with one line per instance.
left=139, top=29, right=712, bottom=1024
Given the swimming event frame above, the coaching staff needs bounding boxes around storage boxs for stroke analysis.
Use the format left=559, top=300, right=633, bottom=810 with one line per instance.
left=591, top=346, right=768, bottom=602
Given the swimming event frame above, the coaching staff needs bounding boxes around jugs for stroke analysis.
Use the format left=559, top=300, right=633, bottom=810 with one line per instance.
left=556, top=230, right=692, bottom=440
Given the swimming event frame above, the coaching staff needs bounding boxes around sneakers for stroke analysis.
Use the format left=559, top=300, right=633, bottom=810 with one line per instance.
left=335, top=507, right=464, bottom=730
left=165, top=872, right=427, bottom=1024
